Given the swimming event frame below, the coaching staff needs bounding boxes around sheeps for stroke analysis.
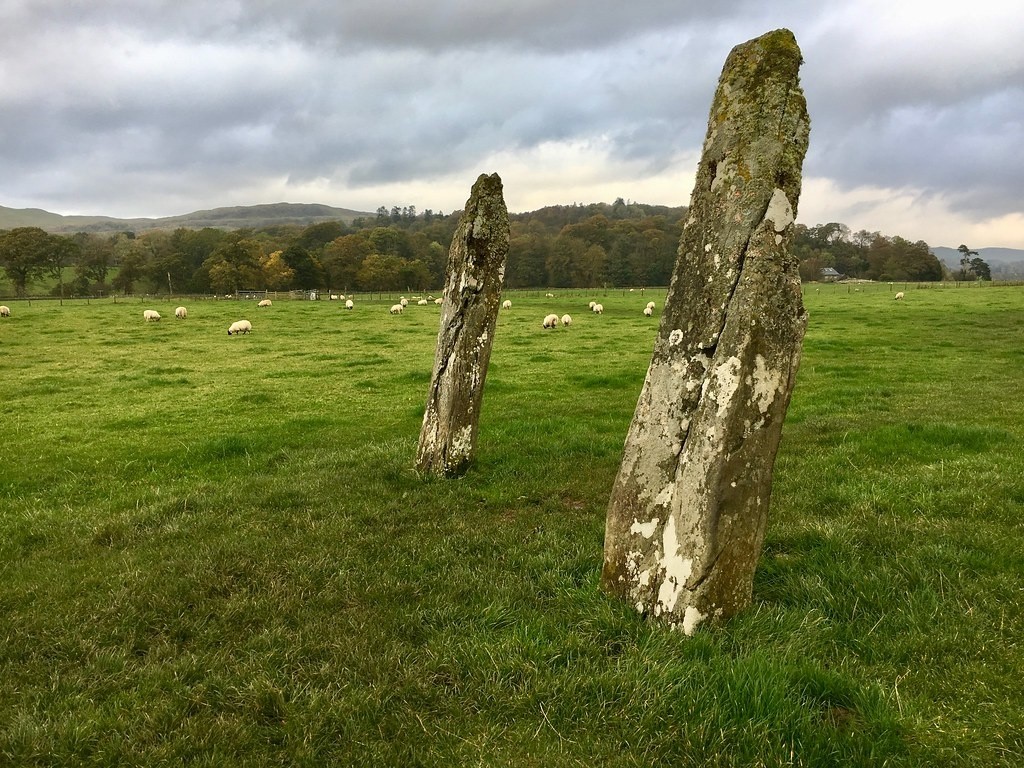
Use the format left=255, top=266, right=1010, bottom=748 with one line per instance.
left=1, top=276, right=915, bottom=337
